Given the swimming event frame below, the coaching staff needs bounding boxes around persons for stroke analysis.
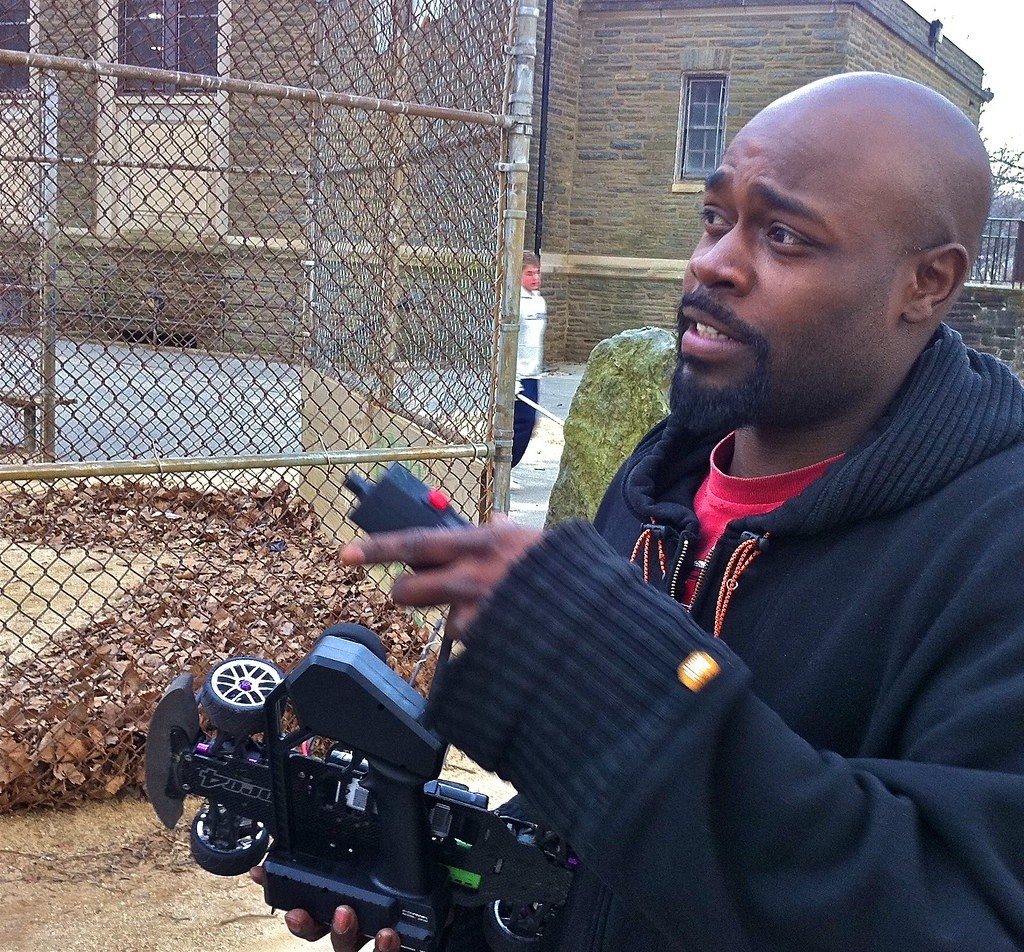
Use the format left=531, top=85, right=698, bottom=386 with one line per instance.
left=491, top=251, right=547, bottom=469
left=250, top=71, right=1024, bottom=952
